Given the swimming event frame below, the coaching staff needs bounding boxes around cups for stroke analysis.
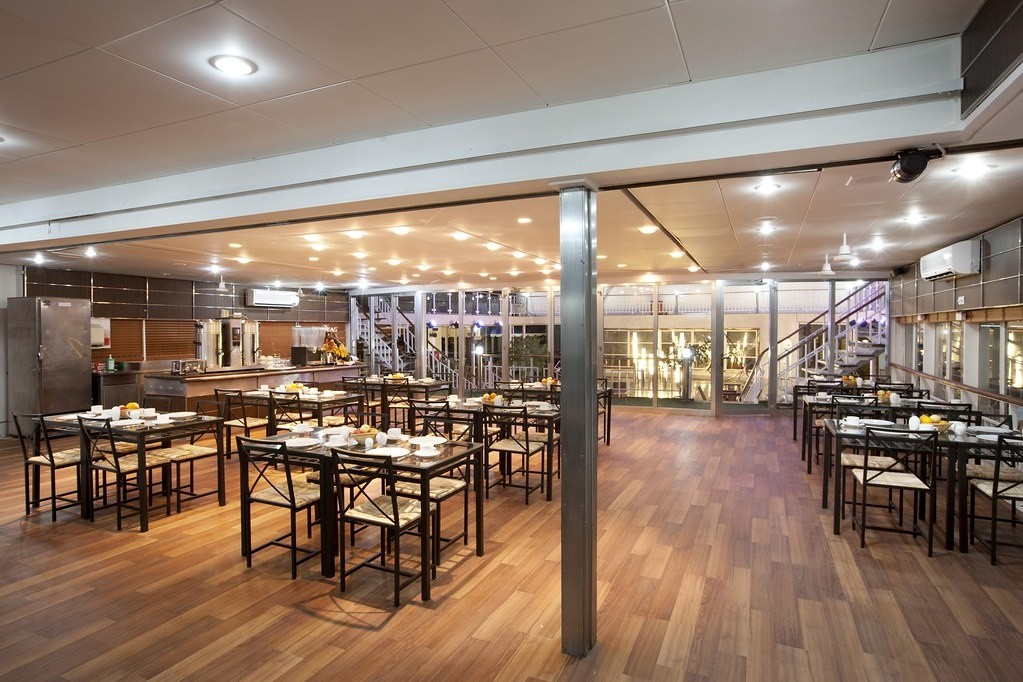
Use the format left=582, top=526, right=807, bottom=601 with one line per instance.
left=535, top=382, right=542, bottom=386
left=540, top=404, right=550, bottom=409
left=466, top=398, right=477, bottom=404
left=953, top=422, right=967, bottom=435
left=260, top=384, right=332, bottom=396
left=844, top=416, right=859, bottom=423
left=864, top=380, right=870, bottom=383
left=261, top=385, right=268, bottom=390
left=919, top=424, right=939, bottom=431
left=296, top=423, right=309, bottom=430
left=329, top=436, right=344, bottom=445
left=388, top=428, right=401, bottom=437
left=447, top=395, right=458, bottom=400
left=511, top=400, right=522, bottom=406
left=420, top=442, right=436, bottom=455
left=91, top=405, right=155, bottom=421
left=816, top=392, right=827, bottom=397
left=309, top=388, right=318, bottom=393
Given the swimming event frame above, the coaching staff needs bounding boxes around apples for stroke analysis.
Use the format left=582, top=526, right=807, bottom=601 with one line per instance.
left=354, top=424, right=378, bottom=434
left=483, top=393, right=496, bottom=403
left=393, top=374, right=403, bottom=378
left=920, top=414, right=947, bottom=424
left=542, top=377, right=558, bottom=385
left=842, top=375, right=855, bottom=385
left=878, top=390, right=891, bottom=396
left=119, top=405, right=128, bottom=410
left=286, top=383, right=303, bottom=388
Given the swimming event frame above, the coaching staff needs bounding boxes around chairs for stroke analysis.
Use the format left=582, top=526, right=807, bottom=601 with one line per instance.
left=8, top=374, right=614, bottom=608
left=794, top=375, right=1023, bottom=565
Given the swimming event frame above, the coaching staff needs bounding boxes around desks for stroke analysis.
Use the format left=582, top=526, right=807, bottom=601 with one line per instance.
left=802, top=394, right=968, bottom=474
left=488, top=379, right=612, bottom=446
left=225, top=387, right=365, bottom=460
left=344, top=376, right=454, bottom=436
left=822, top=419, right=1023, bottom=554
left=793, top=385, right=923, bottom=441
left=241, top=426, right=484, bottom=601
left=31, top=409, right=225, bottom=532
left=410, top=397, right=561, bottom=501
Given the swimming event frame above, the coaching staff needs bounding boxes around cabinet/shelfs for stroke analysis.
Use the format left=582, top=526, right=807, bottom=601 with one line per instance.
left=144, top=361, right=369, bottom=410
left=93, top=369, right=137, bottom=406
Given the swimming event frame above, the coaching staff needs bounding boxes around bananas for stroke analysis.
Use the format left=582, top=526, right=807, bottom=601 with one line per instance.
left=334, top=348, right=347, bottom=358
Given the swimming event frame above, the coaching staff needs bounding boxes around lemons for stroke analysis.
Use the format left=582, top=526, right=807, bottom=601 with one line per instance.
left=126, top=402, right=139, bottom=410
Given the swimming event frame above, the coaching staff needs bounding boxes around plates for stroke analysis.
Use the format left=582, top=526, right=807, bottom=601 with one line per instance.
left=446, top=399, right=553, bottom=411
left=817, top=383, right=871, bottom=386
left=277, top=428, right=448, bottom=457
left=510, top=386, right=543, bottom=389
left=843, top=419, right=1014, bottom=433
left=77, top=411, right=197, bottom=426
left=816, top=396, right=860, bottom=404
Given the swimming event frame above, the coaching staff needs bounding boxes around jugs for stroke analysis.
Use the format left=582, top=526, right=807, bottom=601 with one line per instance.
left=857, top=376, right=863, bottom=387
left=909, top=416, right=920, bottom=431
left=890, top=393, right=899, bottom=405
left=112, top=406, right=121, bottom=421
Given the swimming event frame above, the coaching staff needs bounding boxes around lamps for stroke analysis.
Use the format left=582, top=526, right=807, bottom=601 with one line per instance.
left=847, top=319, right=879, bottom=328
left=889, top=152, right=931, bottom=184
left=427, top=321, right=504, bottom=328
left=475, top=340, right=484, bottom=355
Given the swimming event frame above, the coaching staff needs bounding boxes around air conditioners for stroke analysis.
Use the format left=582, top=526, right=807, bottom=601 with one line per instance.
left=920, top=239, right=980, bottom=282
left=246, top=288, right=299, bottom=308
